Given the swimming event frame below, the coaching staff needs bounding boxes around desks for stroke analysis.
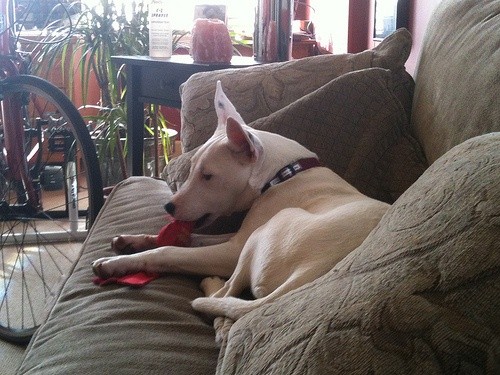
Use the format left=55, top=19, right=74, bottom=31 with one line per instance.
left=17, top=32, right=104, bottom=181
left=110, top=55, right=261, bottom=176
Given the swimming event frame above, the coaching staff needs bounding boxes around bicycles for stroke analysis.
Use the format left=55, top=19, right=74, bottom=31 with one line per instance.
left=1, top=1, right=102, bottom=345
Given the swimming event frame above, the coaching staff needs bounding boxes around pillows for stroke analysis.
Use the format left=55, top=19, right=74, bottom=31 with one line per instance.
left=213, top=129, right=500, bottom=375
left=160, top=67, right=427, bottom=234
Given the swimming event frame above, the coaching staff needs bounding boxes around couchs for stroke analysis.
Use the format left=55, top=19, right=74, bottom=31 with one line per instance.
left=14, top=1, right=500, bottom=374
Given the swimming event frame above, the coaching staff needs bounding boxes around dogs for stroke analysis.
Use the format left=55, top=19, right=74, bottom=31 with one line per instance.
left=91, top=79, right=392, bottom=321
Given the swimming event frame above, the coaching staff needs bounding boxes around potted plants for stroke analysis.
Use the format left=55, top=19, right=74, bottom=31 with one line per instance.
left=27, top=1, right=254, bottom=202
